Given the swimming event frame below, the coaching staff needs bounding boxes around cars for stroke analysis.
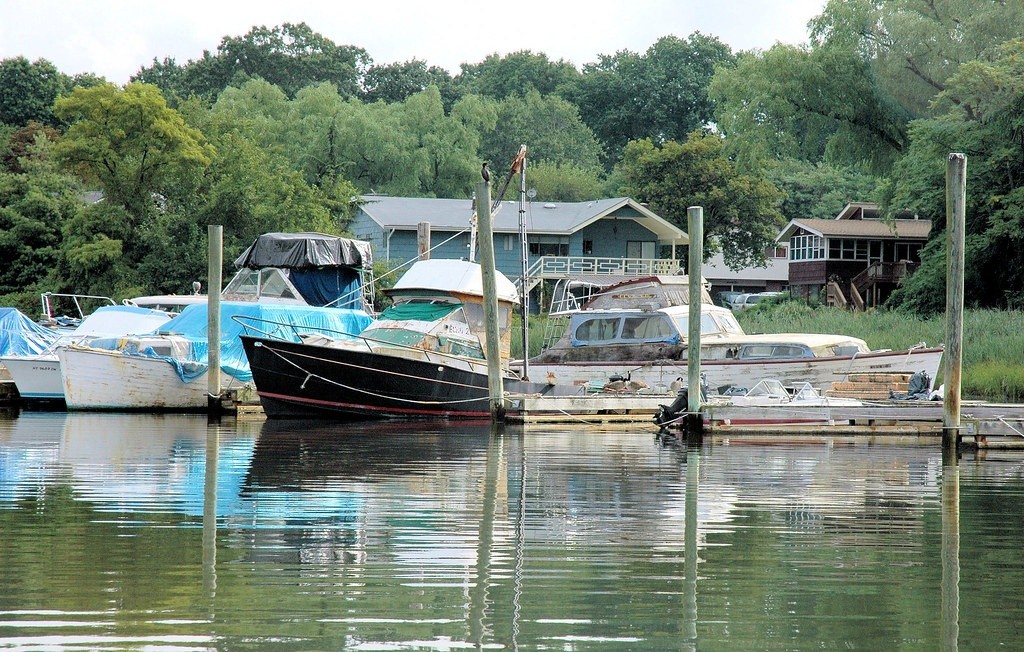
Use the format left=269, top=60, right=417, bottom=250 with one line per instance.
left=713, top=292, right=744, bottom=309
left=732, top=293, right=777, bottom=313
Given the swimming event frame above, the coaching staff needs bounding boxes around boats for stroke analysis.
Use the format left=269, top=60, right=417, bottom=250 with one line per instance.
left=1, top=257, right=942, bottom=432
